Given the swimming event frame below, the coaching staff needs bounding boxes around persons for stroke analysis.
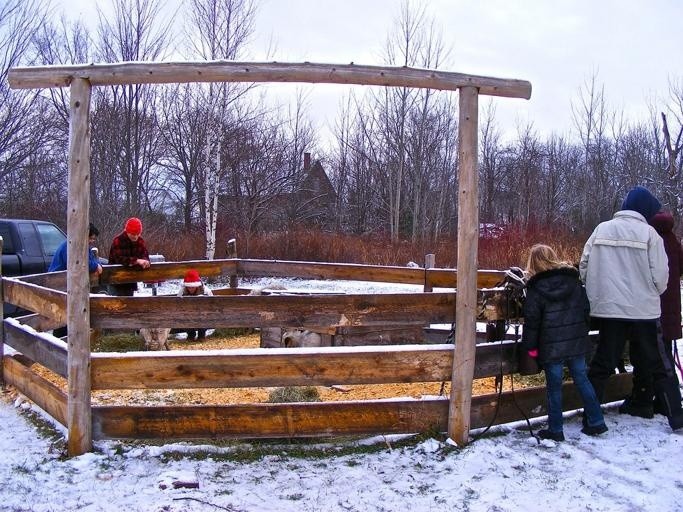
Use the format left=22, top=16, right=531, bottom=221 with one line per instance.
left=521, top=242, right=609, bottom=442
left=107, top=215, right=151, bottom=296
left=48, top=222, right=103, bottom=338
left=176, top=269, right=214, bottom=342
left=579, top=184, right=683, bottom=433
left=619, top=210, right=683, bottom=418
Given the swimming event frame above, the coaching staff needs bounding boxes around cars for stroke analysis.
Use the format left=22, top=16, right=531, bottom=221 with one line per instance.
left=0, top=218, right=111, bottom=316
left=480, top=223, right=504, bottom=239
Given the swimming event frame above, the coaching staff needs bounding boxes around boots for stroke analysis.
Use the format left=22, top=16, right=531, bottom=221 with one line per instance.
left=619, top=371, right=683, bottom=430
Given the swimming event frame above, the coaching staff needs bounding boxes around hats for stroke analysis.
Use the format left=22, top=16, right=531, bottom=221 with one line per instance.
left=621, top=186, right=662, bottom=221
left=125, top=217, right=142, bottom=235
left=182, top=270, right=202, bottom=287
left=648, top=211, right=674, bottom=233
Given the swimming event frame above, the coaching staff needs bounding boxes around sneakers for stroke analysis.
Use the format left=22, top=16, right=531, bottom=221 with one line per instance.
left=537, top=429, right=565, bottom=441
left=581, top=423, right=608, bottom=435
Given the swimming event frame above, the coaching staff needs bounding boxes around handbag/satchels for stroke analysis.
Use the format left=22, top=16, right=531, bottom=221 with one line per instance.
left=518, top=342, right=542, bottom=376
